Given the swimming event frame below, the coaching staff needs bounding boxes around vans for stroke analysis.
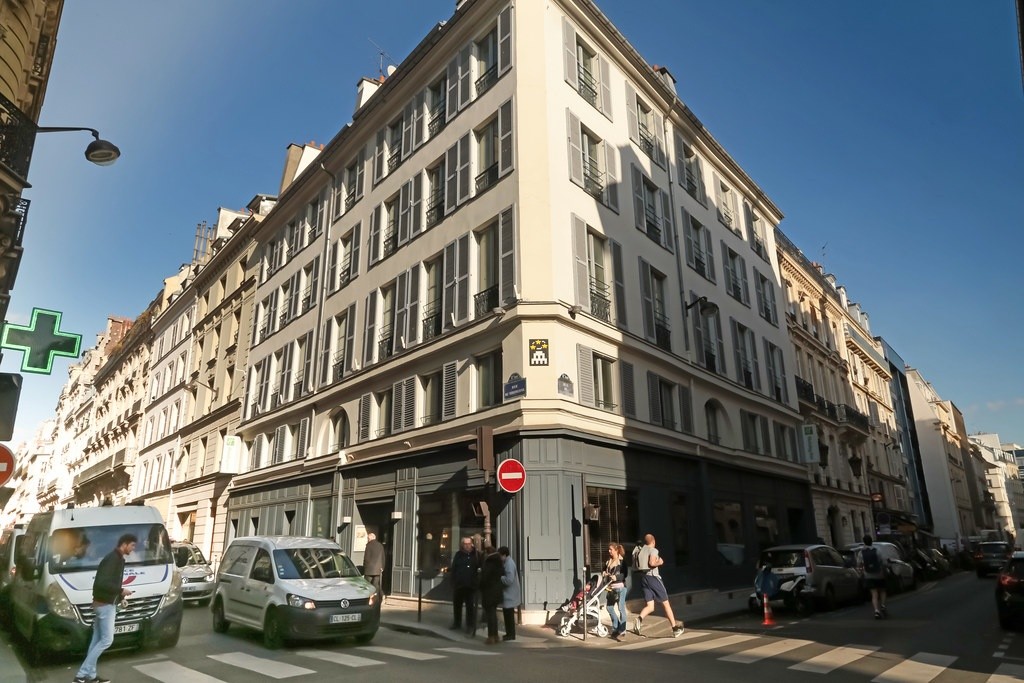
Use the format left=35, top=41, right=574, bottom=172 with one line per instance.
left=209, top=535, right=384, bottom=650
left=0, top=504, right=188, bottom=666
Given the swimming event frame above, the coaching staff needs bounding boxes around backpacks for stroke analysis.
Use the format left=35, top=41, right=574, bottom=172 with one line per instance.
left=631, top=540, right=653, bottom=573
left=862, top=548, right=881, bottom=574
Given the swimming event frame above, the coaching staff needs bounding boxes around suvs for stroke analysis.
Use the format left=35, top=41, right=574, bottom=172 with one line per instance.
left=170, top=540, right=214, bottom=608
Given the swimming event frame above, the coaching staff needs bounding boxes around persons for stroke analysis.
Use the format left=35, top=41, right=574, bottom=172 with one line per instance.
left=72, top=534, right=137, bottom=683
left=451, top=537, right=522, bottom=644
left=857, top=535, right=889, bottom=619
left=363, top=533, right=386, bottom=589
left=602, top=542, right=628, bottom=638
left=634, top=533, right=684, bottom=638
left=572, top=584, right=591, bottom=610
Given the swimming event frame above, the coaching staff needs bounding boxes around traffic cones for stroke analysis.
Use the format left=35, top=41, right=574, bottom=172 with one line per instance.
left=761, top=593, right=781, bottom=624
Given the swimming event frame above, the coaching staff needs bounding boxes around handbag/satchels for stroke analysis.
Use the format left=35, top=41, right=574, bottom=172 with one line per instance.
left=606, top=589, right=618, bottom=602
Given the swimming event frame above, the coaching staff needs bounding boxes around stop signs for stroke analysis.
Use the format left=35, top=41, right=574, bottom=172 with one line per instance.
left=0, top=443, right=16, bottom=487
left=497, top=458, right=526, bottom=493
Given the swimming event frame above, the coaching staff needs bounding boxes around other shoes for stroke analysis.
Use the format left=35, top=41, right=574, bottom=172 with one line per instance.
left=673, top=626, right=685, bottom=638
left=486, top=637, right=499, bottom=645
left=449, top=624, right=461, bottom=630
left=502, top=633, right=515, bottom=640
left=633, top=617, right=643, bottom=637
left=881, top=605, right=890, bottom=617
left=611, top=631, right=618, bottom=639
left=616, top=633, right=625, bottom=641
left=874, top=611, right=881, bottom=618
left=467, top=626, right=475, bottom=637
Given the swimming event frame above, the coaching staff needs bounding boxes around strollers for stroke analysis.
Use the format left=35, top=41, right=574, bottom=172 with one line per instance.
left=556, top=574, right=613, bottom=638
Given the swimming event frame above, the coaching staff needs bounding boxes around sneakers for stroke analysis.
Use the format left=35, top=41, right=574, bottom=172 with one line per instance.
left=72, top=677, right=89, bottom=683
left=90, top=676, right=110, bottom=683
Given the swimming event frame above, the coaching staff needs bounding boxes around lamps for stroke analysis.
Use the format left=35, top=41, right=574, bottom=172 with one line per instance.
left=686, top=294, right=718, bottom=318
left=884, top=439, right=901, bottom=451
left=842, top=516, right=848, bottom=526
left=391, top=511, right=402, bottom=519
left=342, top=516, right=351, bottom=523
left=0, top=122, right=120, bottom=165
left=826, top=514, right=832, bottom=525
left=183, top=380, right=219, bottom=399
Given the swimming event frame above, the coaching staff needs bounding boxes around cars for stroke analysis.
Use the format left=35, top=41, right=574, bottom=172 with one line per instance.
left=973, top=542, right=1011, bottom=577
left=756, top=544, right=865, bottom=608
left=996, top=551, right=1024, bottom=632
left=836, top=539, right=954, bottom=596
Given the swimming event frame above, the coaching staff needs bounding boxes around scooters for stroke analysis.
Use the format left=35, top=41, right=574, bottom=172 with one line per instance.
left=748, top=563, right=813, bottom=614
